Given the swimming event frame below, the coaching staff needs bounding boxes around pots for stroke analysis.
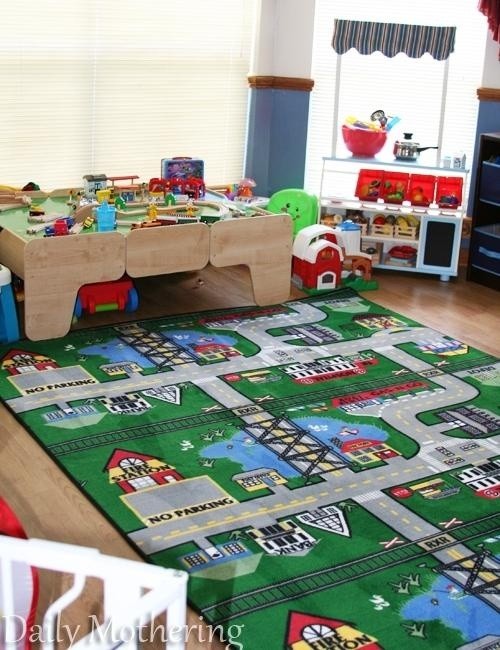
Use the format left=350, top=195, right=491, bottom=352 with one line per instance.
left=393, top=132, right=439, bottom=162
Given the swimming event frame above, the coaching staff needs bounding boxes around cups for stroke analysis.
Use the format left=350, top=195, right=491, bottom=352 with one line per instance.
left=97, top=206, right=117, bottom=233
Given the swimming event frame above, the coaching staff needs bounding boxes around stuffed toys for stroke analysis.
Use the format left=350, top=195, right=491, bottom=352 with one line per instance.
left=72, top=278, right=140, bottom=320
left=17, top=151, right=263, bottom=246
left=265, top=109, right=468, bottom=296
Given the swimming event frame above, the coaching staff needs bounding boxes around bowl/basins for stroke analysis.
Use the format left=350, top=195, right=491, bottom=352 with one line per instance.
left=341, top=124, right=387, bottom=159
